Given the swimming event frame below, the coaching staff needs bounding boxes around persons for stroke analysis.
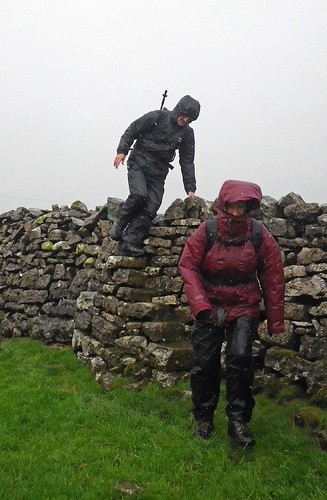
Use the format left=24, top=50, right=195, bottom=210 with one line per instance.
left=178, top=179, right=286, bottom=446
left=109, top=89, right=201, bottom=259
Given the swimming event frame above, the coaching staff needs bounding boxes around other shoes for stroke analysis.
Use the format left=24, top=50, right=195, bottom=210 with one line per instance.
left=110, top=218, right=128, bottom=241
left=118, top=244, right=147, bottom=257
left=228, top=423, right=255, bottom=446
left=196, top=420, right=214, bottom=439
